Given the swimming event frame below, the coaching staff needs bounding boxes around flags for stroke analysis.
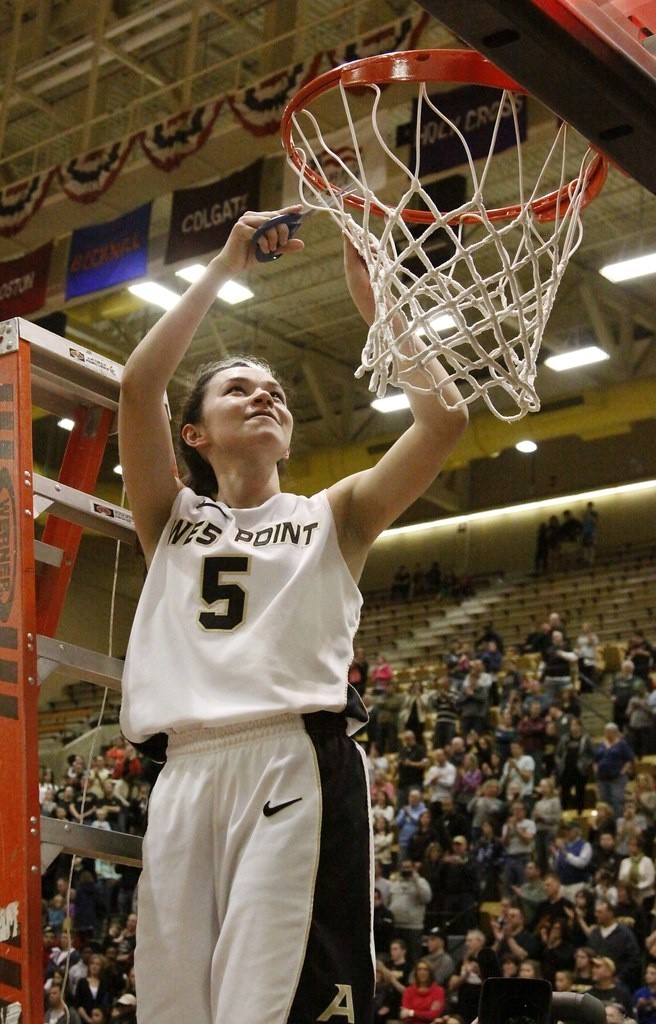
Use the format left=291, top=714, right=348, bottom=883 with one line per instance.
left=63, top=197, right=156, bottom=301
left=407, top=83, right=529, bottom=181
left=280, top=106, right=393, bottom=215
left=162, top=153, right=268, bottom=266
left=0, top=235, right=55, bottom=321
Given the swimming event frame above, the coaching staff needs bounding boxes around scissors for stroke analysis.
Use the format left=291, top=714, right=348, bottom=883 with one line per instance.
left=249, top=178, right=362, bottom=264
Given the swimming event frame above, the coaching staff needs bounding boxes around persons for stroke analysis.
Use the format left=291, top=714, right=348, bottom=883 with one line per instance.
left=112, top=203, right=470, bottom=1024
left=532, top=501, right=600, bottom=576
left=27, top=608, right=656, bottom=1024
left=389, top=558, right=464, bottom=603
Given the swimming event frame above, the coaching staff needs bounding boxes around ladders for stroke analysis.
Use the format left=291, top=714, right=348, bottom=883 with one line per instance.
left=0, top=309, right=178, bottom=1024
